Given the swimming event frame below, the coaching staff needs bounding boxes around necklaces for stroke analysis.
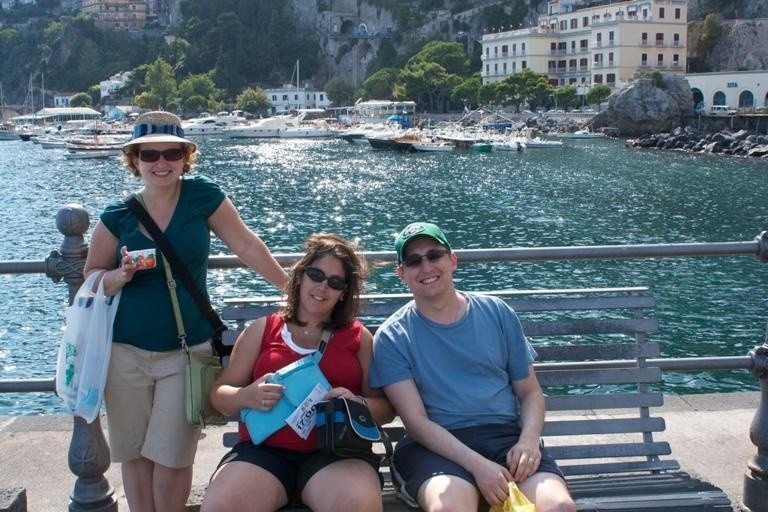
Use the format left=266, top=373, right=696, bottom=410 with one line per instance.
left=302, top=326, right=312, bottom=336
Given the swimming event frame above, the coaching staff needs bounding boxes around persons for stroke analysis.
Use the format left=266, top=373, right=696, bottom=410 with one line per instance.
left=368, top=222, right=575, bottom=512
left=81, top=110, right=291, bottom=512
left=198, top=232, right=397, bottom=511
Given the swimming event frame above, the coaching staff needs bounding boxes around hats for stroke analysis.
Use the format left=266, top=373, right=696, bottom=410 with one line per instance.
left=121, top=109, right=197, bottom=158
left=393, top=221, right=451, bottom=269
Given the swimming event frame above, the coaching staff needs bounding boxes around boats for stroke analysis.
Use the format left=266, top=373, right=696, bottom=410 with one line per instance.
left=181, top=111, right=226, bottom=135
left=281, top=126, right=341, bottom=137
left=0, top=122, right=132, bottom=159
left=367, top=106, right=565, bottom=153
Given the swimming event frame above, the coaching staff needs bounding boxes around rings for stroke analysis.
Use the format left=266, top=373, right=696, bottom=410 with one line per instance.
left=528, top=457, right=534, bottom=462
left=262, top=399, right=265, bottom=407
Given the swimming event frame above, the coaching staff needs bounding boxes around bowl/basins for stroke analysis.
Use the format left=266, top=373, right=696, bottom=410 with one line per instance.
left=126, top=249, right=158, bottom=272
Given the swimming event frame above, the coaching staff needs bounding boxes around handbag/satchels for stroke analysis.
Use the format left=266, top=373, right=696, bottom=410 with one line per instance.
left=210, top=332, right=234, bottom=364
left=239, top=352, right=334, bottom=447
left=182, top=348, right=229, bottom=430
left=310, top=396, right=382, bottom=461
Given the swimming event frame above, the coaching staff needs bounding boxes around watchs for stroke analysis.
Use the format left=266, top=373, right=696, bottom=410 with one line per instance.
left=357, top=393, right=368, bottom=406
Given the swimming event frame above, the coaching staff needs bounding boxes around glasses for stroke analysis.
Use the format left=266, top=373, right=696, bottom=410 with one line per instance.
left=137, top=148, right=186, bottom=162
left=303, top=264, right=347, bottom=291
left=401, top=249, right=451, bottom=268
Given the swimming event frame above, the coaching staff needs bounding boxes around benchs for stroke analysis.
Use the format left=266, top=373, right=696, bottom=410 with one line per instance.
left=222, top=287, right=733, bottom=511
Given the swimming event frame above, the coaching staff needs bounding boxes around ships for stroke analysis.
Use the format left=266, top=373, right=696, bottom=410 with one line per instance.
left=229, top=114, right=304, bottom=138
